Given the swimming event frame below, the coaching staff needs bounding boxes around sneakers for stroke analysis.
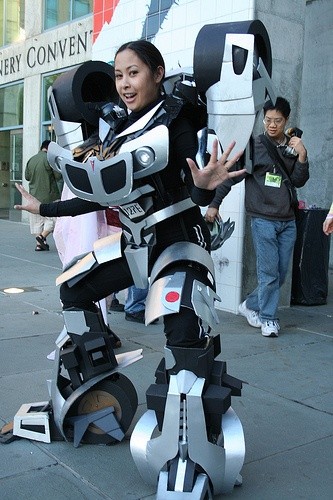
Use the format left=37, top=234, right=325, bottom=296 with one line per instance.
left=261, top=320, right=281, bottom=337
left=126, top=310, right=159, bottom=324
left=239, top=300, right=261, bottom=328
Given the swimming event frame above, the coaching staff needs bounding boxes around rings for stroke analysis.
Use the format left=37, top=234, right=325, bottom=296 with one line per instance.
left=224, top=164, right=230, bottom=172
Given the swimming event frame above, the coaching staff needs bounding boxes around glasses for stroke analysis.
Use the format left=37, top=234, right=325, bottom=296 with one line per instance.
left=262, top=117, right=286, bottom=125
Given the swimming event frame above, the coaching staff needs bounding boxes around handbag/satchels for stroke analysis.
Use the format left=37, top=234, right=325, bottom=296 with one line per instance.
left=288, top=185, right=305, bottom=219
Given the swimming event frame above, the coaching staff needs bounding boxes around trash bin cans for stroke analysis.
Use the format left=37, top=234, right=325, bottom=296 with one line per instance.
left=290, top=201, right=331, bottom=307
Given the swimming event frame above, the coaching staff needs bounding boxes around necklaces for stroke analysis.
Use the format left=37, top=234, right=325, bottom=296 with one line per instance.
left=204, top=96, right=310, bottom=337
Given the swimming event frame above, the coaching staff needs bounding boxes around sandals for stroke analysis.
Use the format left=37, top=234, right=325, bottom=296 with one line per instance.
left=36, top=235, right=49, bottom=251
left=34, top=245, right=45, bottom=251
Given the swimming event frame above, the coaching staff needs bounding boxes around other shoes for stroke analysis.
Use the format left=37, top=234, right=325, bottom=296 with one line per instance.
left=109, top=299, right=125, bottom=311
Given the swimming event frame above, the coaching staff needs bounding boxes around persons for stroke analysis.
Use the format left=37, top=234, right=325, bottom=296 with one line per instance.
left=0, top=20, right=286, bottom=500
left=24, top=135, right=163, bottom=325
left=324, top=202, right=333, bottom=236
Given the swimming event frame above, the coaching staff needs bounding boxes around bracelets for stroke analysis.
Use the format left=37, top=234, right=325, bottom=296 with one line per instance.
left=327, top=213, right=333, bottom=218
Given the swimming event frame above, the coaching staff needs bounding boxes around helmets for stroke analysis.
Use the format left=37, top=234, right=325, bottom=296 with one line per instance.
left=203, top=215, right=235, bottom=251
